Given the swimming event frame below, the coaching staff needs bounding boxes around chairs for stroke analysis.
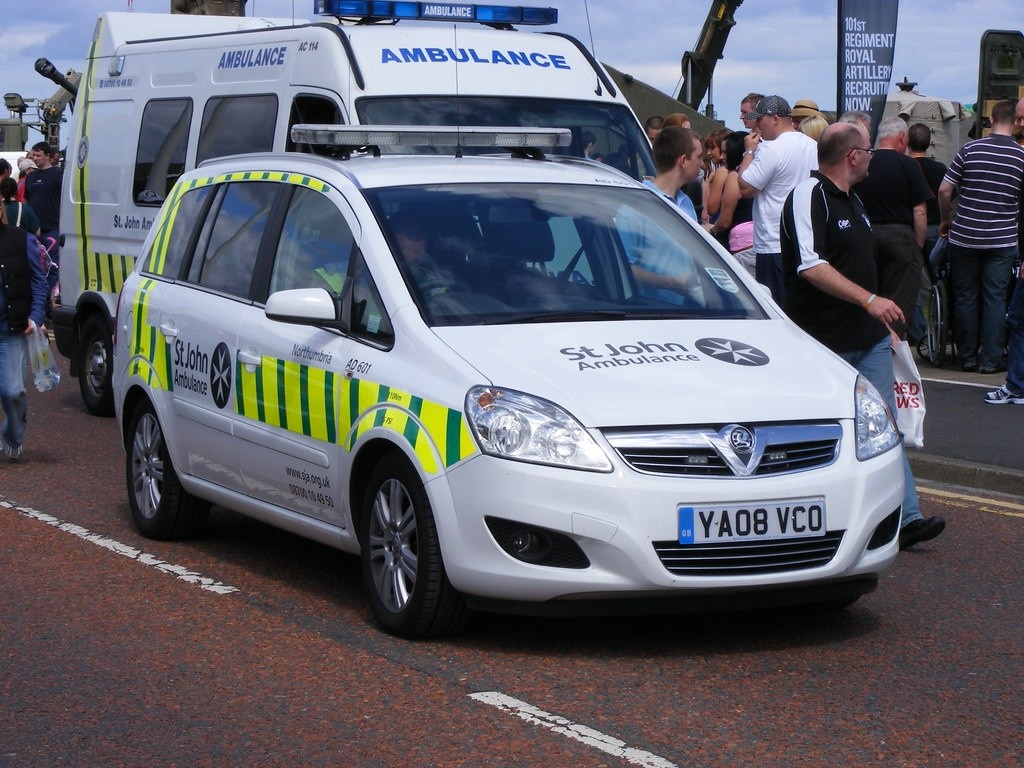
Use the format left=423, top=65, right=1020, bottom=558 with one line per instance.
left=482, top=203, right=556, bottom=282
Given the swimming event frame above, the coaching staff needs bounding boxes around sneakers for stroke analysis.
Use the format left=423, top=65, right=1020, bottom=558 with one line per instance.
left=3, top=433, right=23, bottom=460
left=985, top=381, right=1024, bottom=404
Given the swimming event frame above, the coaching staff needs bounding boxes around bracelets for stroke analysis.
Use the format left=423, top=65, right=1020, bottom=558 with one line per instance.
left=863, top=294, right=877, bottom=310
left=708, top=224, right=718, bottom=236
left=743, top=150, right=754, bottom=156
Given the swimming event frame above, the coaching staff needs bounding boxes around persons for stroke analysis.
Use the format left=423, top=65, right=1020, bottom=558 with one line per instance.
left=780, top=123, right=946, bottom=551
left=136, top=189, right=165, bottom=204
left=615, top=126, right=705, bottom=309
left=311, top=213, right=472, bottom=337
left=582, top=93, right=1024, bottom=404
left=0, top=141, right=62, bottom=462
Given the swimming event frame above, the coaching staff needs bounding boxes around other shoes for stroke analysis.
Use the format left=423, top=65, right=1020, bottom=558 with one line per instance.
left=961, top=357, right=1008, bottom=373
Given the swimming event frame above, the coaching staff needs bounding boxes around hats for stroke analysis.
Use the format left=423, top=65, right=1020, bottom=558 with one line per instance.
left=746, top=95, right=792, bottom=120
left=792, top=99, right=829, bottom=121
left=19, top=159, right=38, bottom=171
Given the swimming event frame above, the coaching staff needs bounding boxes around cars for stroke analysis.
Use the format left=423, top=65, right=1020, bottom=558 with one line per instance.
left=112, top=123, right=907, bottom=640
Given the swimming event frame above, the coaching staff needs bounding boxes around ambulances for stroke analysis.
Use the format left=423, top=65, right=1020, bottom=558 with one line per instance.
left=50, top=0, right=662, bottom=418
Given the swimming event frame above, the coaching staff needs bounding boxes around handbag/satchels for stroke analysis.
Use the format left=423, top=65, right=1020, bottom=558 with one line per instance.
left=892, top=341, right=926, bottom=450
left=26, top=319, right=62, bottom=393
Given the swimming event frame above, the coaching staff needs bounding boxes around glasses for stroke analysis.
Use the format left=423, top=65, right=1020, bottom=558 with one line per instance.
left=847, top=147, right=875, bottom=159
